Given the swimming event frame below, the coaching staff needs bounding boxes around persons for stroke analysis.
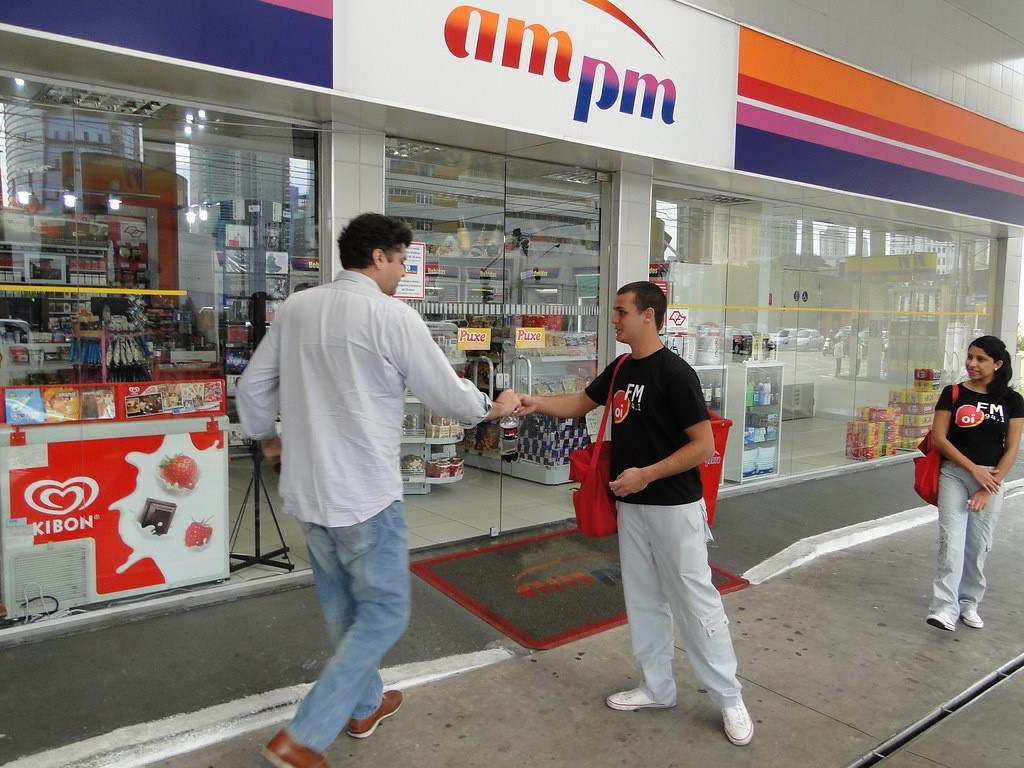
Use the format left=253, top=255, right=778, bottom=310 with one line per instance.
left=926, top=335, right=1024, bottom=631
left=234, top=212, right=521, bottom=768
left=834, top=335, right=843, bottom=375
left=512, top=281, right=754, bottom=745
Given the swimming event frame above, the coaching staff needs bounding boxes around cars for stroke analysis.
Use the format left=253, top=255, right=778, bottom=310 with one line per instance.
left=696, top=325, right=890, bottom=355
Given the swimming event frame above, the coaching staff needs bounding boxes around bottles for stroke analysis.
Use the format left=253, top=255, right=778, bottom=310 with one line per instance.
left=501, top=415, right=518, bottom=463
left=754, top=340, right=758, bottom=361
left=746, top=377, right=780, bottom=406
left=471, top=222, right=502, bottom=256
left=701, top=383, right=721, bottom=409
left=745, top=413, right=778, bottom=426
left=457, top=215, right=471, bottom=256
left=226, top=352, right=251, bottom=375
left=144, top=297, right=216, bottom=357
left=768, top=341, right=776, bottom=360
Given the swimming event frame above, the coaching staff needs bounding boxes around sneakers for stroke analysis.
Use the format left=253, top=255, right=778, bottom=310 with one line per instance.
left=607, top=687, right=675, bottom=711
left=260, top=729, right=327, bottom=768
left=926, top=613, right=955, bottom=633
left=722, top=698, right=754, bottom=745
left=347, top=689, right=403, bottom=739
left=961, top=610, right=983, bottom=628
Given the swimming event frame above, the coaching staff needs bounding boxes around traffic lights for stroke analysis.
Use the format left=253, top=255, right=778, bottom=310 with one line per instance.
left=481, top=286, right=494, bottom=303
left=520, top=238, right=530, bottom=257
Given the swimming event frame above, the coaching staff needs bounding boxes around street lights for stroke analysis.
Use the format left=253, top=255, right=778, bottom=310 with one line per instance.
left=514, top=243, right=563, bottom=304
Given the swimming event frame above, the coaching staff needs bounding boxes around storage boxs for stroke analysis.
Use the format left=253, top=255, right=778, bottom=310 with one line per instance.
left=847, top=369, right=941, bottom=462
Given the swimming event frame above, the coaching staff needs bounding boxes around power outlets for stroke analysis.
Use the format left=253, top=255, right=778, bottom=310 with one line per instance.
left=398, top=320, right=464, bottom=496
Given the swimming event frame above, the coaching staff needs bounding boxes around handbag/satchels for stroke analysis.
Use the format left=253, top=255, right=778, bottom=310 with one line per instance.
left=912, top=430, right=941, bottom=507
left=568, top=440, right=618, bottom=538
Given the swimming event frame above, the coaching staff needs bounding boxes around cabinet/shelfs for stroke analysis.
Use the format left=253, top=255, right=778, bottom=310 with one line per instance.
left=723, top=362, right=784, bottom=482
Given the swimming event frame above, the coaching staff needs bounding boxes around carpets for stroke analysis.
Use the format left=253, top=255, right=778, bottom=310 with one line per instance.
left=407, top=523, right=750, bottom=651
